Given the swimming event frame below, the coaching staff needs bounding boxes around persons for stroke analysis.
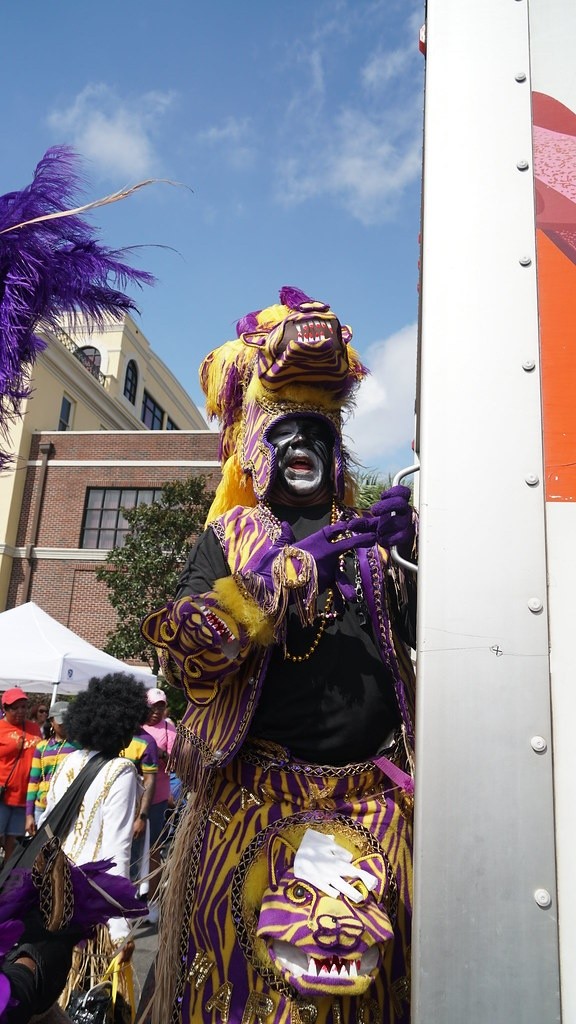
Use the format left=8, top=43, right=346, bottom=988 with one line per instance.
left=0, top=674, right=188, bottom=1024
left=144, top=287, right=419, bottom=1024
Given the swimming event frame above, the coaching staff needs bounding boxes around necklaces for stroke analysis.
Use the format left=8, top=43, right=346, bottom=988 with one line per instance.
left=258, top=500, right=348, bottom=661
left=40, top=737, right=67, bottom=792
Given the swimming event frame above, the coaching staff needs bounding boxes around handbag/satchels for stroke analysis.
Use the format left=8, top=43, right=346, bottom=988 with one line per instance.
left=0, top=786, right=6, bottom=801
left=0, top=835, right=34, bottom=888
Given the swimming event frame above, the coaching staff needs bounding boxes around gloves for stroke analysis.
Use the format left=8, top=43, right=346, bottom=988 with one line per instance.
left=237, top=521, right=376, bottom=616
left=347, top=485, right=416, bottom=584
left=5, top=911, right=95, bottom=1015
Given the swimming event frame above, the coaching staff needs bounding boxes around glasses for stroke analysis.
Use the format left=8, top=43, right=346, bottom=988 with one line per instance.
left=39, top=709, right=49, bottom=714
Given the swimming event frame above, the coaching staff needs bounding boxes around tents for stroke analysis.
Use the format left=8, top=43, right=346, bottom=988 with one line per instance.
left=0, top=602, right=158, bottom=717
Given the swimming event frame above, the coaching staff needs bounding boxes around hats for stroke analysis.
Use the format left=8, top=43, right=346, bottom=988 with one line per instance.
left=47, top=701, right=69, bottom=724
left=146, top=688, right=167, bottom=706
left=2, top=687, right=28, bottom=705
